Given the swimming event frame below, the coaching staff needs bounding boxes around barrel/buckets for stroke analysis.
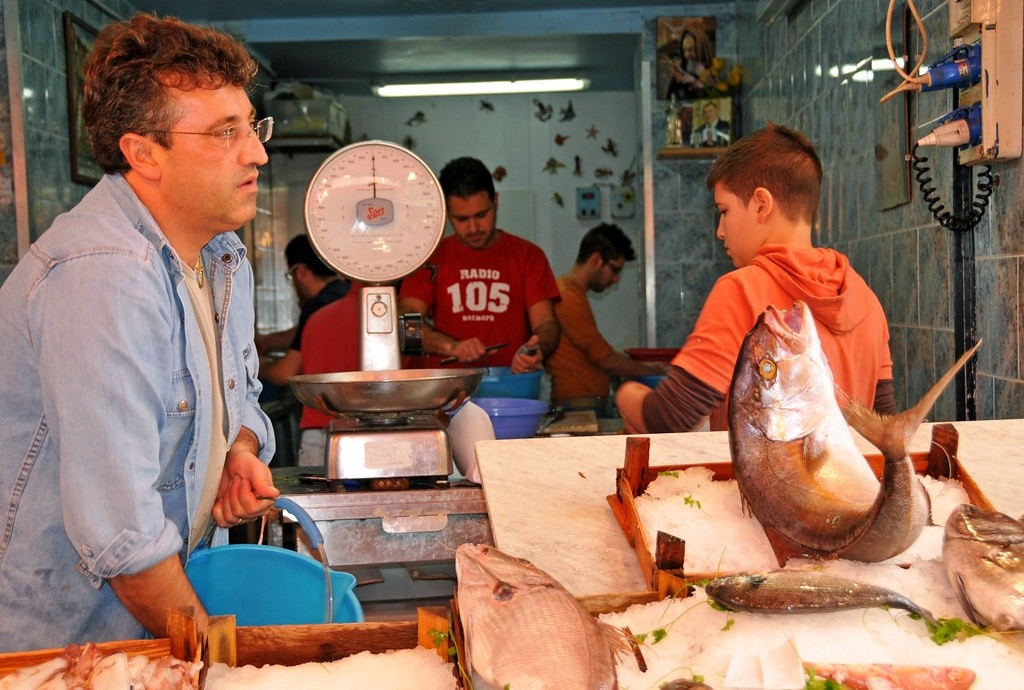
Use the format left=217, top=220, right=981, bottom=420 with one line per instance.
left=141, top=496, right=364, bottom=643
left=623, top=347, right=683, bottom=374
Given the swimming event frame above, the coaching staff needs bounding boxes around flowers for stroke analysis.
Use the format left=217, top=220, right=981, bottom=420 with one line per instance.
left=700, top=59, right=742, bottom=98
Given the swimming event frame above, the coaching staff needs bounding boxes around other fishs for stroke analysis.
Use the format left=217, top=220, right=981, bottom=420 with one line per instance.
left=943, top=502, right=1024, bottom=632
left=453, top=542, right=661, bottom=689
left=706, top=571, right=946, bottom=632
left=726, top=300, right=982, bottom=569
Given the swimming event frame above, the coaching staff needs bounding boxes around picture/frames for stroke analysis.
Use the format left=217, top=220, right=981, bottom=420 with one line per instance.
left=63, top=9, right=102, bottom=185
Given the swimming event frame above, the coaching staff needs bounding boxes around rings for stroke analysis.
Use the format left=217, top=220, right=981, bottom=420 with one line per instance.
left=233, top=519, right=242, bottom=525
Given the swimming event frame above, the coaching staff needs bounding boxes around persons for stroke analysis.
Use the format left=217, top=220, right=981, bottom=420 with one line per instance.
left=255, top=234, right=412, bottom=465
left=399, top=158, right=562, bottom=373
left=0, top=15, right=280, bottom=650
left=544, top=223, right=665, bottom=415
left=692, top=102, right=729, bottom=146
left=615, top=123, right=897, bottom=435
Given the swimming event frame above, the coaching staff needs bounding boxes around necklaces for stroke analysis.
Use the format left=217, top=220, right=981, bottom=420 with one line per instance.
left=191, top=253, right=204, bottom=288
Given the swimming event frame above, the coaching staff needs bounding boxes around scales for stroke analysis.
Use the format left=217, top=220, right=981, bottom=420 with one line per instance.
left=283, top=137, right=488, bottom=490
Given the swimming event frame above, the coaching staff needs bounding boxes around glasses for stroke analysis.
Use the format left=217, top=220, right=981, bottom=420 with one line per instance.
left=285, top=263, right=303, bottom=281
left=140, top=116, right=273, bottom=151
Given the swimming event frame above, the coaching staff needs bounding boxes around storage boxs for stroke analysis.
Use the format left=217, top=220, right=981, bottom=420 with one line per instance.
left=268, top=96, right=347, bottom=141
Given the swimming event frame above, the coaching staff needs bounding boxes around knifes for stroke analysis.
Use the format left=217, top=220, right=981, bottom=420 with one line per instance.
left=439, top=340, right=509, bottom=366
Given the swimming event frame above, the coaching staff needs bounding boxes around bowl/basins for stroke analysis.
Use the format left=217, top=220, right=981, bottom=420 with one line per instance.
left=471, top=398, right=549, bottom=438
left=641, top=375, right=665, bottom=390
left=472, top=367, right=544, bottom=399
